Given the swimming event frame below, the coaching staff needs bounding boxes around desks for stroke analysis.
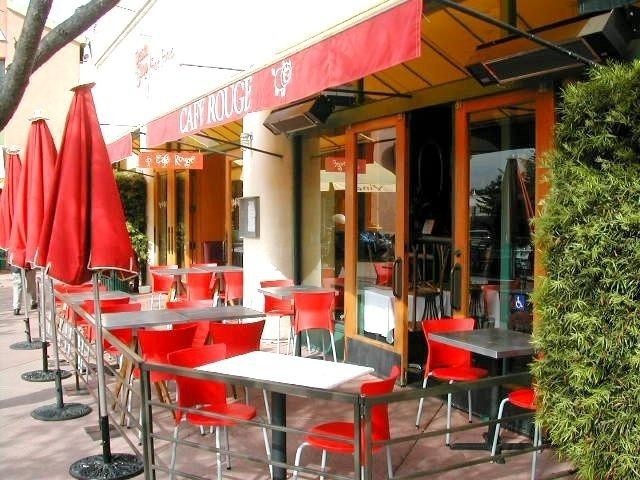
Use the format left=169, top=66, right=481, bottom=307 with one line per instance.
left=426, top=331, right=546, bottom=465
left=191, top=349, right=375, bottom=480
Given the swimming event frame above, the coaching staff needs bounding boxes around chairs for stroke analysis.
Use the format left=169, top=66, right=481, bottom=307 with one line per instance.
left=415, top=319, right=488, bottom=446
left=127, top=322, right=265, bottom=446
left=168, top=343, right=274, bottom=479
left=45, top=257, right=536, bottom=423
left=291, top=366, right=399, bottom=480
left=491, top=389, right=557, bottom=478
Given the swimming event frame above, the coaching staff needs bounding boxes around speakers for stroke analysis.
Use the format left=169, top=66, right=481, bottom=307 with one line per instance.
left=262, top=95, right=333, bottom=135
left=463, top=9, right=633, bottom=87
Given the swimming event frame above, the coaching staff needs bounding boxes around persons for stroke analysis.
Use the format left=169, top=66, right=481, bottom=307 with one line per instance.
left=12, top=266, right=37, bottom=315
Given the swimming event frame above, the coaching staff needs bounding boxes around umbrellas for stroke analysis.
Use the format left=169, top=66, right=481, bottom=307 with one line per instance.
left=0, top=147, right=31, bottom=342
left=34, top=82, right=139, bottom=463
left=8, top=110, right=58, bottom=372
